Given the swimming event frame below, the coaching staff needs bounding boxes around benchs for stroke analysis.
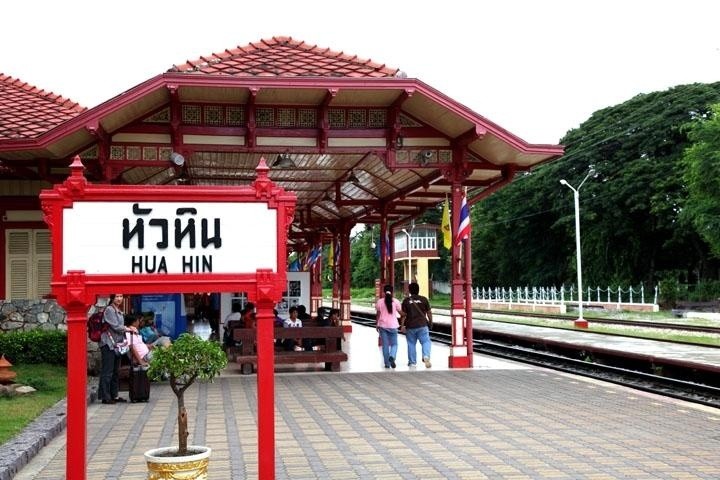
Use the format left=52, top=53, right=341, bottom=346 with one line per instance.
left=228, top=318, right=348, bottom=374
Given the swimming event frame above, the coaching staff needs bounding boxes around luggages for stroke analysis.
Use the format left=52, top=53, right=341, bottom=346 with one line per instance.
left=128, top=330, right=151, bottom=403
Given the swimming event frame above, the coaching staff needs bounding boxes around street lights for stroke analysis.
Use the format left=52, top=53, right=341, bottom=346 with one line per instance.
left=401, top=224, right=416, bottom=296
left=559, top=169, right=596, bottom=328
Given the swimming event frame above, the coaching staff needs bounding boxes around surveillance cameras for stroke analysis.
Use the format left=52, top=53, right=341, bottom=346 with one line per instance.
left=422, top=150, right=433, bottom=158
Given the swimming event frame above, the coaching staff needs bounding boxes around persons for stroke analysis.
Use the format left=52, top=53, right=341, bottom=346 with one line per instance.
left=375, top=284, right=408, bottom=369
left=136, top=315, right=155, bottom=344
left=400, top=283, right=433, bottom=370
left=120, top=314, right=176, bottom=383
left=99, top=294, right=128, bottom=403
left=143, top=311, right=161, bottom=338
left=195, top=293, right=347, bottom=351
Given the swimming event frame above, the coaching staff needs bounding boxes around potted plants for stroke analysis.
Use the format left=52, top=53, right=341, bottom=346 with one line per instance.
left=143, top=332, right=228, bottom=480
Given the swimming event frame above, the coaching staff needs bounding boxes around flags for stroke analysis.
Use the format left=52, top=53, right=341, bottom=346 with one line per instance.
left=384, top=227, right=390, bottom=262
left=288, top=238, right=342, bottom=274
left=375, top=228, right=381, bottom=261
left=454, top=190, right=473, bottom=251
left=439, top=193, right=451, bottom=254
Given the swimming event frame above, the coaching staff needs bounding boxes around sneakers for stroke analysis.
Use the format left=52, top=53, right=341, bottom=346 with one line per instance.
left=102, top=396, right=126, bottom=404
left=408, top=355, right=432, bottom=368
left=385, top=356, right=397, bottom=368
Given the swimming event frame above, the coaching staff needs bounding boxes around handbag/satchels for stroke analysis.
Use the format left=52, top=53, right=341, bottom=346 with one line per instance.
left=113, top=339, right=129, bottom=356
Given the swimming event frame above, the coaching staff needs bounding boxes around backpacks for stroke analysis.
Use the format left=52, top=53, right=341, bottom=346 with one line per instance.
left=88, top=305, right=118, bottom=342
